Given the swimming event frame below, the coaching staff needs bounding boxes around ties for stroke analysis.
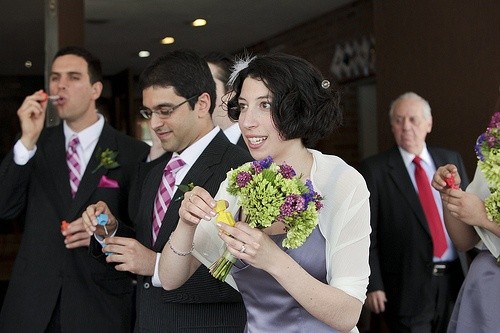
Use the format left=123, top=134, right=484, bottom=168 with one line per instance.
left=152, top=156, right=186, bottom=247
left=413, top=156, right=448, bottom=259
left=66, top=135, right=80, bottom=200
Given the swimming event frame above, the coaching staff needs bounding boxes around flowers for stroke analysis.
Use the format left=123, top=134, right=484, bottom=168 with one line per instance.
left=475, top=112, right=500, bottom=224
left=91, top=145, right=119, bottom=174
left=207, top=154, right=328, bottom=284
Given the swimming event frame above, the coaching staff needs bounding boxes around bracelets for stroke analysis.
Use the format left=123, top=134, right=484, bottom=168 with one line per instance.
left=169, top=233, right=195, bottom=256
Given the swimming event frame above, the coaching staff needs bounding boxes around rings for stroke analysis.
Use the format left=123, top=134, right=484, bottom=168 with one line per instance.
left=240, top=242, right=246, bottom=252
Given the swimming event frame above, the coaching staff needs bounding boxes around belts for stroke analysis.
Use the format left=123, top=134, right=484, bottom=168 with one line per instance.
left=433, top=257, right=460, bottom=274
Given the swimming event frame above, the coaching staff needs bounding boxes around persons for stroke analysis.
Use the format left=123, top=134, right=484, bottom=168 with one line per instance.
left=0, top=46, right=151, bottom=333
left=83, top=52, right=254, bottom=333
left=431, top=113, right=500, bottom=333
left=158, top=51, right=373, bottom=333
left=359, top=92, right=472, bottom=333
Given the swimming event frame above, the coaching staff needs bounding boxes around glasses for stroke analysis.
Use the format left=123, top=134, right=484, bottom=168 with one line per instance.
left=140, top=94, right=199, bottom=120
